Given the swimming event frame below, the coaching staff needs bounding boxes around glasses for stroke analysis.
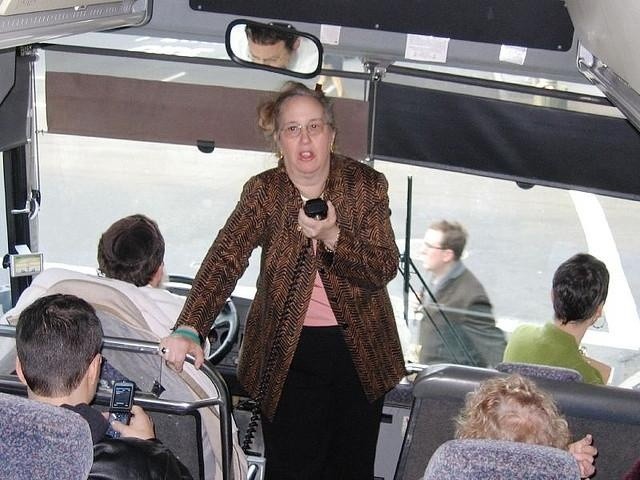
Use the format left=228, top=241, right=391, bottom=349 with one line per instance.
left=273, top=124, right=323, bottom=136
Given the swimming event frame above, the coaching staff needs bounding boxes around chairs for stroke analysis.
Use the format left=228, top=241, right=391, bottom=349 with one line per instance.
left=2, top=279, right=247, bottom=480
left=499, top=363, right=581, bottom=384
left=423, top=439, right=580, bottom=480
left=0, top=394, right=93, bottom=480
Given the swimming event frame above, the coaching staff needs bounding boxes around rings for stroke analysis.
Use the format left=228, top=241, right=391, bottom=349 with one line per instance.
left=297, top=226, right=302, bottom=231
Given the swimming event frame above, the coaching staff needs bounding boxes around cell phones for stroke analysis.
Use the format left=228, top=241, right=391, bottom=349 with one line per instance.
left=106, top=380, right=136, bottom=437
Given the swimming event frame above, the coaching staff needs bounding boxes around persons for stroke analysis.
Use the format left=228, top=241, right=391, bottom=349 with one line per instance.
left=453, top=373, right=598, bottom=480
left=245, top=23, right=301, bottom=69
left=503, top=253, right=610, bottom=385
left=96, top=213, right=210, bottom=360
left=407, top=218, right=508, bottom=370
left=15, top=292, right=196, bottom=480
left=156, top=84, right=407, bottom=480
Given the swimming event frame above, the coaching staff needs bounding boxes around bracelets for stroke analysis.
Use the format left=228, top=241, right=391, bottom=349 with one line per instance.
left=174, top=328, right=200, bottom=345
